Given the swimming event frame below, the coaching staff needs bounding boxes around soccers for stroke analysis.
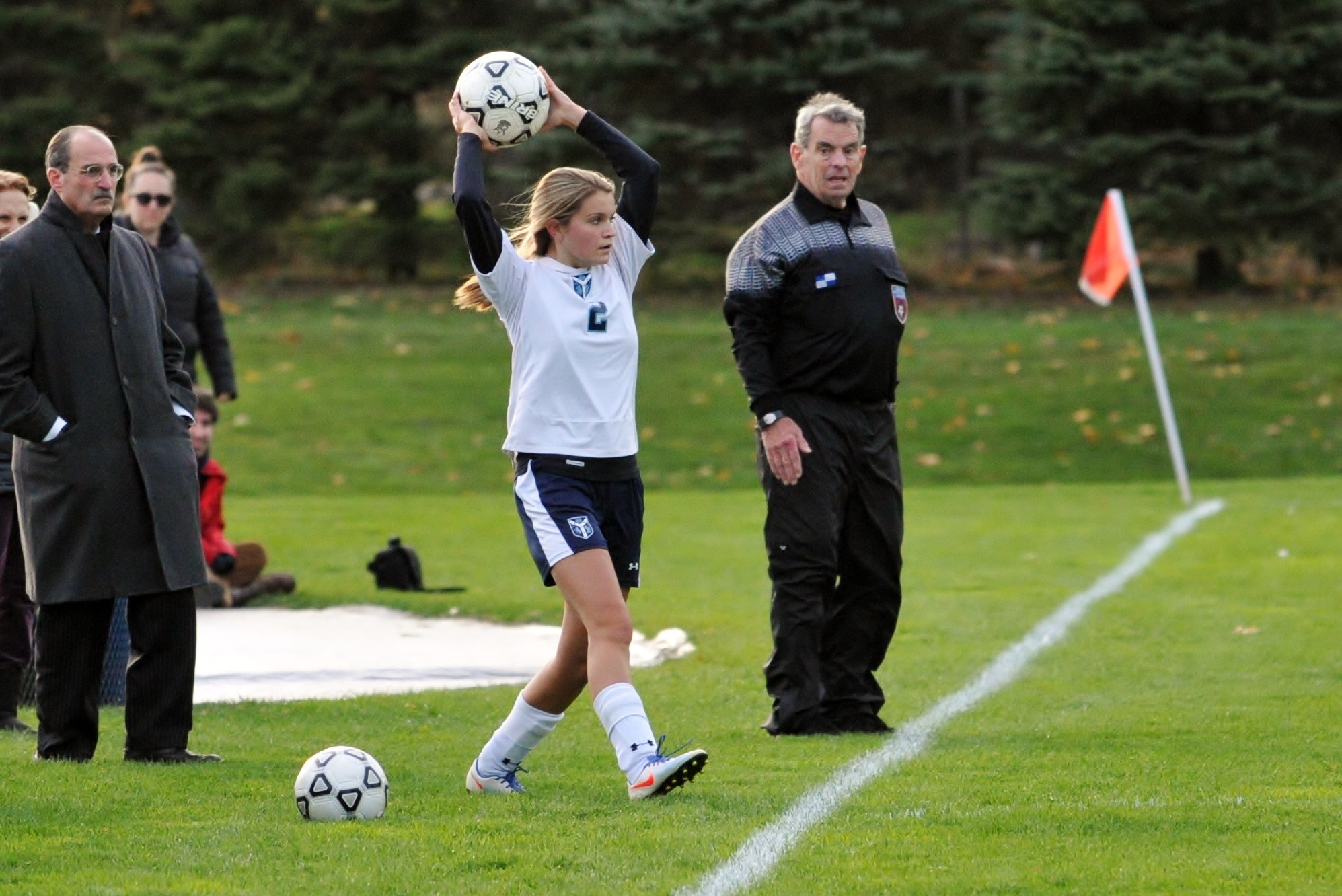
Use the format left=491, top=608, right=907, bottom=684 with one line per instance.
left=453, top=48, right=550, bottom=148
left=294, top=743, right=388, bottom=823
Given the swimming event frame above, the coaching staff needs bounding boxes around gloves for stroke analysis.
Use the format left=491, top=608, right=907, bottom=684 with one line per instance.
left=212, top=553, right=235, bottom=576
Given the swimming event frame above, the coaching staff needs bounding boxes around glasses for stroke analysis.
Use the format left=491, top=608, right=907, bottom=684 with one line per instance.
left=136, top=192, right=172, bottom=208
left=67, top=163, right=123, bottom=183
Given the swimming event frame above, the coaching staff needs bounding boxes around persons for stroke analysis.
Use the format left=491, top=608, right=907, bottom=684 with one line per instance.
left=0, top=170, right=39, bottom=733
left=448, top=64, right=708, bottom=801
left=113, top=144, right=238, bottom=403
left=0, top=122, right=226, bottom=766
left=724, top=91, right=909, bottom=734
left=191, top=392, right=296, bottom=609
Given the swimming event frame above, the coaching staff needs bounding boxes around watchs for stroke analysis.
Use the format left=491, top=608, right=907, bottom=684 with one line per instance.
left=758, top=410, right=785, bottom=430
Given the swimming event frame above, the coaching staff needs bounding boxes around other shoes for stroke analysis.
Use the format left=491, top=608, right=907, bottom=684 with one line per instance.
left=2, top=716, right=38, bottom=735
left=779, top=712, right=896, bottom=735
left=124, top=748, right=225, bottom=766
left=259, top=574, right=295, bottom=595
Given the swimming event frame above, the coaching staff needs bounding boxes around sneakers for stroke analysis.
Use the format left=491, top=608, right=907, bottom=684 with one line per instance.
left=627, top=749, right=709, bottom=802
left=466, top=758, right=527, bottom=794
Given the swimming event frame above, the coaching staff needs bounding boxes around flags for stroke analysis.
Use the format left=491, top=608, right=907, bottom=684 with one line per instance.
left=1072, top=192, right=1133, bottom=304
